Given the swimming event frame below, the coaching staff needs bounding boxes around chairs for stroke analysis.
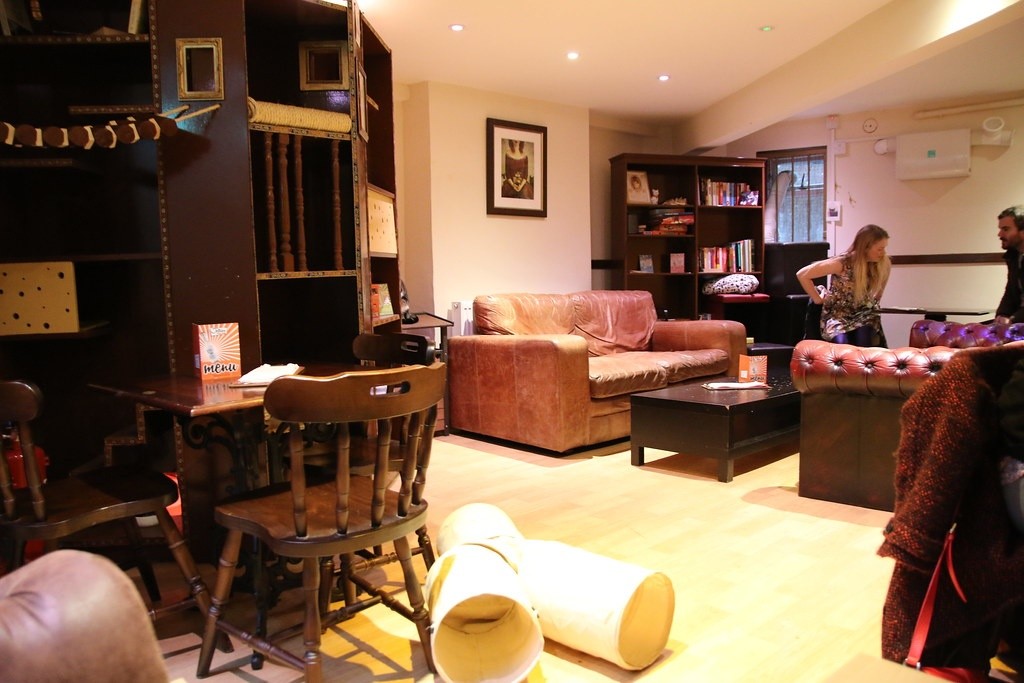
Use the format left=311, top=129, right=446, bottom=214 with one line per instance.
left=195, top=360, right=448, bottom=683
left=718, top=241, right=830, bottom=362
left=885, top=344, right=1024, bottom=683
left=0, top=380, right=233, bottom=655
left=271, top=334, right=438, bottom=634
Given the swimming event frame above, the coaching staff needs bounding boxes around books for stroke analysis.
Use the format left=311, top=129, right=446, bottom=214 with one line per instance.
left=639, top=254, right=654, bottom=272
left=698, top=238, right=755, bottom=272
left=637, top=209, right=696, bottom=236
left=670, top=253, right=685, bottom=273
left=699, top=175, right=758, bottom=206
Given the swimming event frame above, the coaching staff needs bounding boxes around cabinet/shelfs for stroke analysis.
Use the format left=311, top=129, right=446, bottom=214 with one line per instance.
left=608, top=153, right=768, bottom=321
left=401, top=312, right=455, bottom=435
left=0, top=0, right=401, bottom=560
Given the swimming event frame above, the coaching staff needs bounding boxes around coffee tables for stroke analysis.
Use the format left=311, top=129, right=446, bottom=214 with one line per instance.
left=630, top=374, right=802, bottom=483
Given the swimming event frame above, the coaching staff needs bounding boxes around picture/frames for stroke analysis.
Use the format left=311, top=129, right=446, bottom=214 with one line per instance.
left=826, top=201, right=841, bottom=222
left=485, top=118, right=547, bottom=218
left=298, top=40, right=350, bottom=91
left=356, top=58, right=369, bottom=143
left=627, top=170, right=652, bottom=205
left=175, top=37, right=223, bottom=101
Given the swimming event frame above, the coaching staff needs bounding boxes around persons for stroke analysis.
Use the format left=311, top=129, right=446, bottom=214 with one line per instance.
left=370, top=283, right=379, bottom=319
left=979, top=205, right=1024, bottom=326
left=796, top=226, right=893, bottom=349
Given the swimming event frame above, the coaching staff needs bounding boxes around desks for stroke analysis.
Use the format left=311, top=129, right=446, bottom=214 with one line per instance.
left=89, top=366, right=401, bottom=669
left=747, top=341, right=794, bottom=370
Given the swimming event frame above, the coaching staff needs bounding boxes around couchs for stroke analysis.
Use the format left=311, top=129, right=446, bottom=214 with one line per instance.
left=448, top=290, right=747, bottom=452
left=789, top=318, right=1024, bottom=513
